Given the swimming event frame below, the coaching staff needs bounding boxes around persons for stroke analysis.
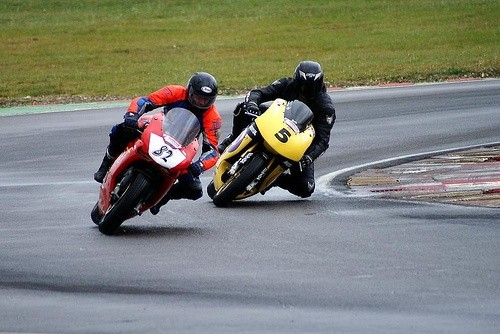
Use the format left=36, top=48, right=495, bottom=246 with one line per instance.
left=94, top=72, right=221, bottom=216
left=217, top=60, right=337, bottom=198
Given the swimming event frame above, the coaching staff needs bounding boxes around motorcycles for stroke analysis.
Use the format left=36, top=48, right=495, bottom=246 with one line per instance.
left=207, top=98, right=316, bottom=207
left=91, top=107, right=198, bottom=235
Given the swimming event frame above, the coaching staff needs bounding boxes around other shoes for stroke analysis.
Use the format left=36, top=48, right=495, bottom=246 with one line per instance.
left=217, top=133, right=235, bottom=155
left=260, top=182, right=278, bottom=196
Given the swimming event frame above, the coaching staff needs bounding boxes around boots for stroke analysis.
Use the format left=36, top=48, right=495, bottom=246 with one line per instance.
left=93, top=145, right=119, bottom=183
left=150, top=183, right=173, bottom=216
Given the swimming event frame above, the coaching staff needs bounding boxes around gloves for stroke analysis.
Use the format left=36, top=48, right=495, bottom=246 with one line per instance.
left=246, top=104, right=259, bottom=122
left=300, top=154, right=314, bottom=169
left=123, top=111, right=139, bottom=132
left=186, top=162, right=202, bottom=177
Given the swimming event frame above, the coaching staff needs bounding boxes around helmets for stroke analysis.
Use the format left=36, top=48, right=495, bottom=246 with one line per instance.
left=292, top=60, right=324, bottom=99
left=186, top=72, right=218, bottom=115
